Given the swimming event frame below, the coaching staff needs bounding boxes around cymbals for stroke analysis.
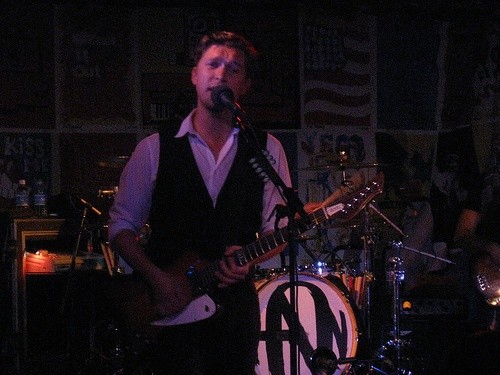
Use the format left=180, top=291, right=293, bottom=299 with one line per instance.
left=295, top=235, right=315, bottom=243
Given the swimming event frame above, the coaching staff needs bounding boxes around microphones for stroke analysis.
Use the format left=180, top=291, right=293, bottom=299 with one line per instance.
left=211, top=85, right=238, bottom=113
left=76, top=197, right=101, bottom=215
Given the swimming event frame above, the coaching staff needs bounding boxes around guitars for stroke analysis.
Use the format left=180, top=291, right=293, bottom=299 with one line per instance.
left=125, top=170, right=383, bottom=326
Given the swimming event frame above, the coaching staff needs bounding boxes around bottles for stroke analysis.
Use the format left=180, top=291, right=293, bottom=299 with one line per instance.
left=15, top=180, right=29, bottom=206
left=34, top=179, right=47, bottom=218
left=16, top=203, right=33, bottom=219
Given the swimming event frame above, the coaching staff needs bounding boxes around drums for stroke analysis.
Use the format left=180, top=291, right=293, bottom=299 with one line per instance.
left=250, top=266, right=357, bottom=375
left=332, top=244, right=372, bottom=276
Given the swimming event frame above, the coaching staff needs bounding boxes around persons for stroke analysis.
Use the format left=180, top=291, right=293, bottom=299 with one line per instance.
left=387, top=152, right=500, bottom=375
left=108, top=30, right=293, bottom=375
